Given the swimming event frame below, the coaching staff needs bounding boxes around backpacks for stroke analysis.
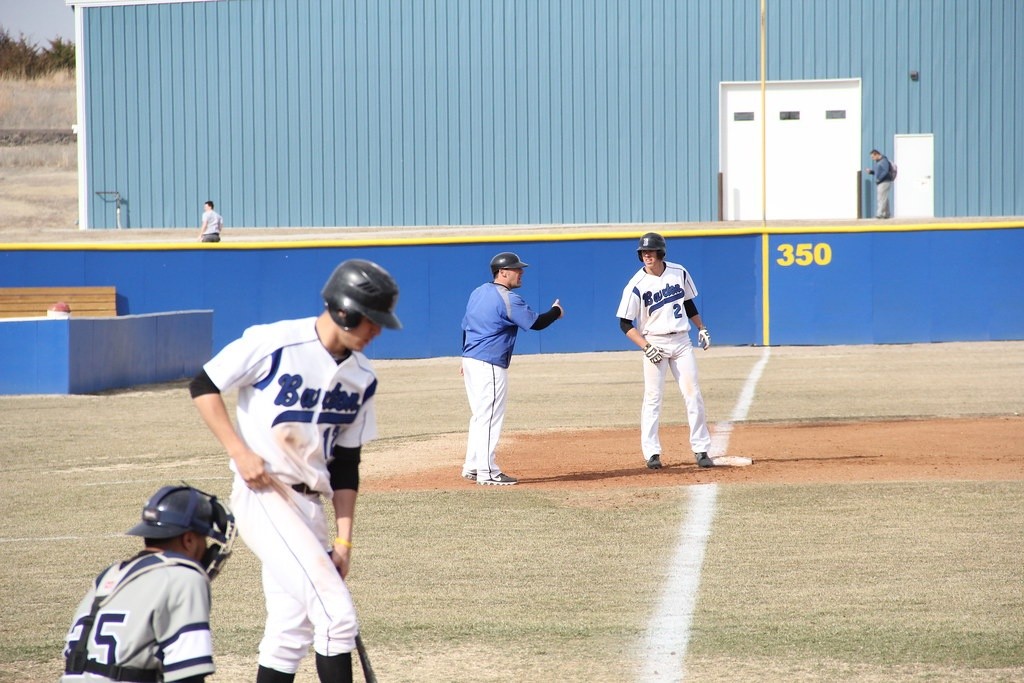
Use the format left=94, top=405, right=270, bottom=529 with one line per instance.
left=888, top=160, right=897, bottom=181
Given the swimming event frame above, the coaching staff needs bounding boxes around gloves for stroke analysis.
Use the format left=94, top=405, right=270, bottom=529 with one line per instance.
left=697, top=327, right=711, bottom=350
left=642, top=343, right=663, bottom=364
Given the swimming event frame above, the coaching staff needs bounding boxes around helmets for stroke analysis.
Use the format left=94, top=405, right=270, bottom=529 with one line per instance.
left=125, top=480, right=214, bottom=537
left=321, top=258, right=402, bottom=331
left=636, top=232, right=666, bottom=262
left=489, top=252, right=528, bottom=274
left=52, top=303, right=71, bottom=312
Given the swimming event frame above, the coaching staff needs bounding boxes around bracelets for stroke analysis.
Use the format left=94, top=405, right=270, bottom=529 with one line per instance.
left=335, top=538, right=352, bottom=548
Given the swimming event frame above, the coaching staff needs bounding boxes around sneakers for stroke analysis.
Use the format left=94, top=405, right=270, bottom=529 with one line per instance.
left=694, top=452, right=714, bottom=466
left=647, top=454, right=663, bottom=469
left=477, top=472, right=517, bottom=485
left=463, top=470, right=477, bottom=480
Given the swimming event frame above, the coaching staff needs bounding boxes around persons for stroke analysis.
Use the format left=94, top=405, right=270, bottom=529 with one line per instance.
left=61, top=484, right=238, bottom=683
left=199, top=201, right=223, bottom=242
left=461, top=252, right=563, bottom=485
left=616, top=232, right=714, bottom=468
left=865, top=150, right=891, bottom=218
left=188, top=260, right=402, bottom=683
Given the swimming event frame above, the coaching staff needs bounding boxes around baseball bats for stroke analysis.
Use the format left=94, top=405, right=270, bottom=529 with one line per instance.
left=327, top=547, right=378, bottom=683
